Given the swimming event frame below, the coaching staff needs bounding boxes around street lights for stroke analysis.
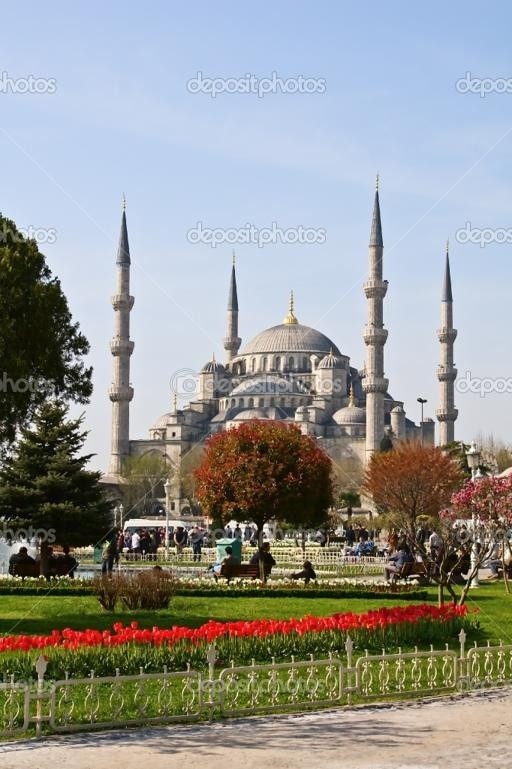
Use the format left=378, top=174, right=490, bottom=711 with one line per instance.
left=112, top=503, right=125, bottom=532
left=466, top=440, right=486, bottom=588
left=163, top=478, right=172, bottom=562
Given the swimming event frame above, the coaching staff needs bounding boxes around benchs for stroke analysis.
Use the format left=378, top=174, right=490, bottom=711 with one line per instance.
left=214, top=564, right=272, bottom=583
left=8, top=560, right=75, bottom=581
left=391, top=559, right=472, bottom=585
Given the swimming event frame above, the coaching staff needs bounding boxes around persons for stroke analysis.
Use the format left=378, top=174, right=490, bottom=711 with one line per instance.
left=207, top=547, right=240, bottom=586
left=291, top=561, right=316, bottom=582
left=339, top=523, right=375, bottom=555
left=223, top=523, right=258, bottom=542
left=384, top=523, right=512, bottom=583
left=99, top=536, right=116, bottom=582
left=7, top=545, right=79, bottom=580
left=116, top=525, right=212, bottom=562
left=251, top=542, right=276, bottom=582
